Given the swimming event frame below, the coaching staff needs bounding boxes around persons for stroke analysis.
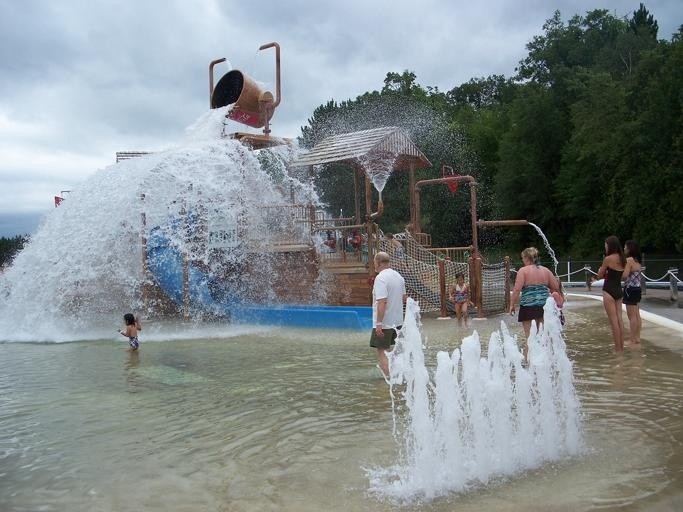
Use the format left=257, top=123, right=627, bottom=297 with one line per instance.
left=370, top=251, right=408, bottom=380
left=118, top=312, right=141, bottom=351
left=353, top=227, right=406, bottom=269
left=449, top=273, right=474, bottom=327
left=598, top=236, right=641, bottom=355
left=591, top=275, right=599, bottom=287
left=510, top=247, right=565, bottom=368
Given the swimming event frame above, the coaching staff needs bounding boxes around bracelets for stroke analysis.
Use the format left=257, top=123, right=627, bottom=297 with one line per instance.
left=120, top=331, right=123, bottom=334
left=376, top=323, right=382, bottom=326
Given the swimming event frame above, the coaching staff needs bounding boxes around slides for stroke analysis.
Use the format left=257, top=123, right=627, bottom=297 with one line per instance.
left=145, top=230, right=373, bottom=330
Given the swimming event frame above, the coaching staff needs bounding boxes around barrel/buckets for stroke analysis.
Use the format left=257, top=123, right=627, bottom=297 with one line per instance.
left=210, top=69, right=275, bottom=129
